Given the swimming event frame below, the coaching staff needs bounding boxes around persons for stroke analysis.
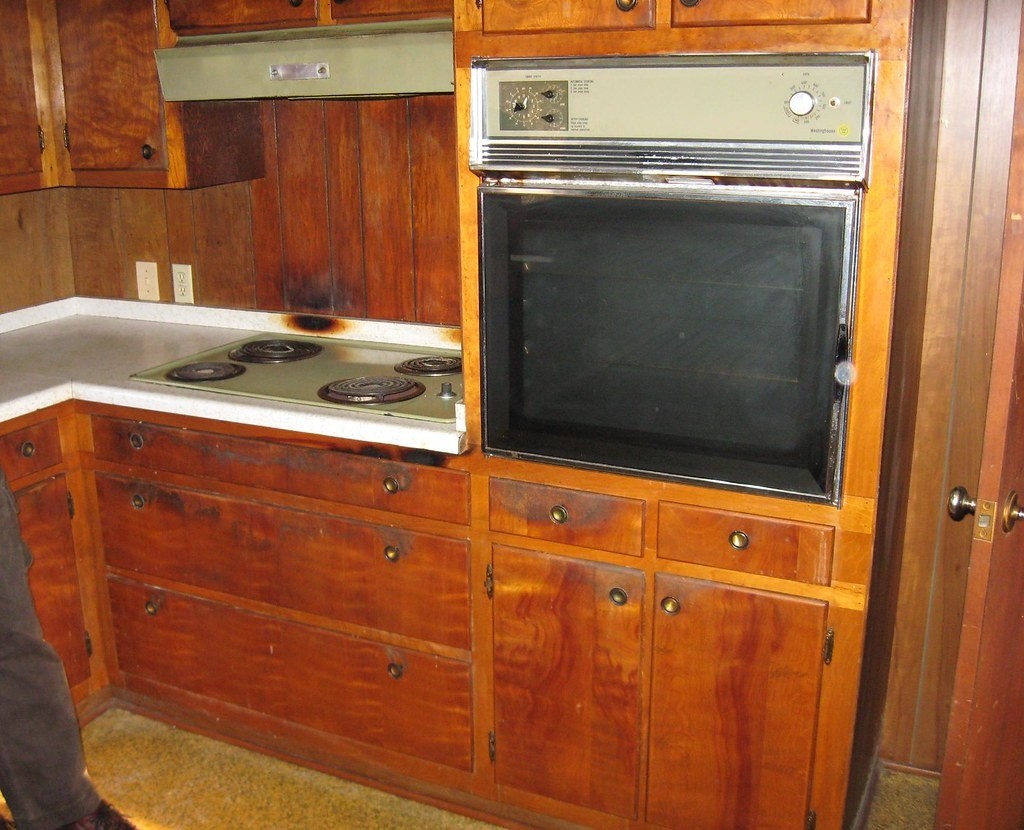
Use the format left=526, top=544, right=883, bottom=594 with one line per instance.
left=0, top=461, right=142, bottom=830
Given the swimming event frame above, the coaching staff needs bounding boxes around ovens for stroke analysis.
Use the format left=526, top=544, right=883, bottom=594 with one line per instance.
left=465, top=47, right=876, bottom=512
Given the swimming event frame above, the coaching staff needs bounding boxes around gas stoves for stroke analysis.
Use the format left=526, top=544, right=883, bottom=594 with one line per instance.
left=130, top=333, right=463, bottom=429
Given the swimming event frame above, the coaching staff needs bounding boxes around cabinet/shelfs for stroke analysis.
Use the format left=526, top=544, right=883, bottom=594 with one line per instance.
left=1, top=400, right=112, bottom=728
left=450, top=1, right=946, bottom=829
left=0, top=0, right=266, bottom=195
left=164, top=0, right=454, bottom=38
left=76, top=401, right=478, bottom=815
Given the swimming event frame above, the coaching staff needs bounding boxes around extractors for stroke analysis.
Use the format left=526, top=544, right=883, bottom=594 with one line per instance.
left=152, top=18, right=457, bottom=103
